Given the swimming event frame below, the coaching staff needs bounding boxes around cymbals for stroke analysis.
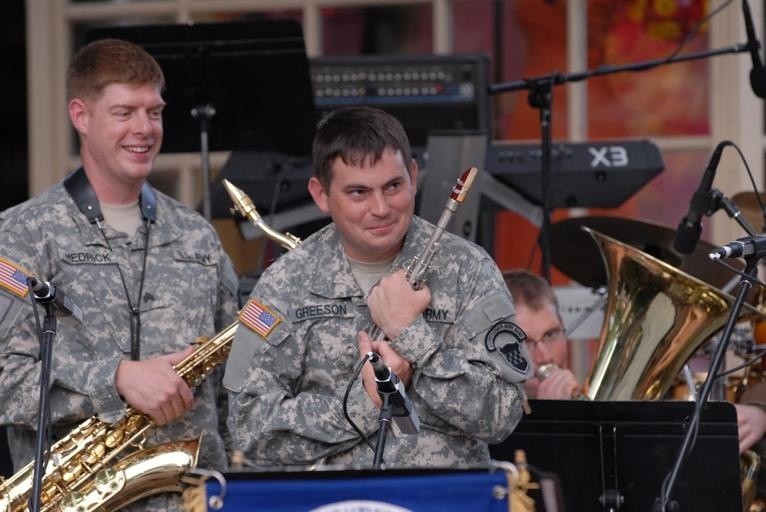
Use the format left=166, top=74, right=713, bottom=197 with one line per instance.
left=539, top=216, right=765, bottom=317
left=730, top=192, right=765, bottom=235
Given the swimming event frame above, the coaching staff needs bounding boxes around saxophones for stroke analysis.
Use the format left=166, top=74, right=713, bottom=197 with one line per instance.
left=0, top=179, right=303, bottom=512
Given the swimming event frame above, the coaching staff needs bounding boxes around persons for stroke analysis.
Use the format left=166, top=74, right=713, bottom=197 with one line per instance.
left=1, top=39, right=242, bottom=512
left=223, top=106, right=530, bottom=470
left=731, top=377, right=764, bottom=456
left=485, top=267, right=581, bottom=511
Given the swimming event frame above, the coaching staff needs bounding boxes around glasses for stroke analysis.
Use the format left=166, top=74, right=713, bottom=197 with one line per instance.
left=524, top=329, right=559, bottom=351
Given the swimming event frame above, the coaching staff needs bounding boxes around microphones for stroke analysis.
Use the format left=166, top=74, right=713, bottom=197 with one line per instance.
left=739, top=0, right=766, bottom=101
left=672, top=139, right=729, bottom=254
left=366, top=345, right=423, bottom=436
left=706, top=235, right=765, bottom=262
left=25, top=275, right=86, bottom=324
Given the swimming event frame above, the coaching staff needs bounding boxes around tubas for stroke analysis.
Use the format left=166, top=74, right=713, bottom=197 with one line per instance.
left=491, top=227, right=766, bottom=511
